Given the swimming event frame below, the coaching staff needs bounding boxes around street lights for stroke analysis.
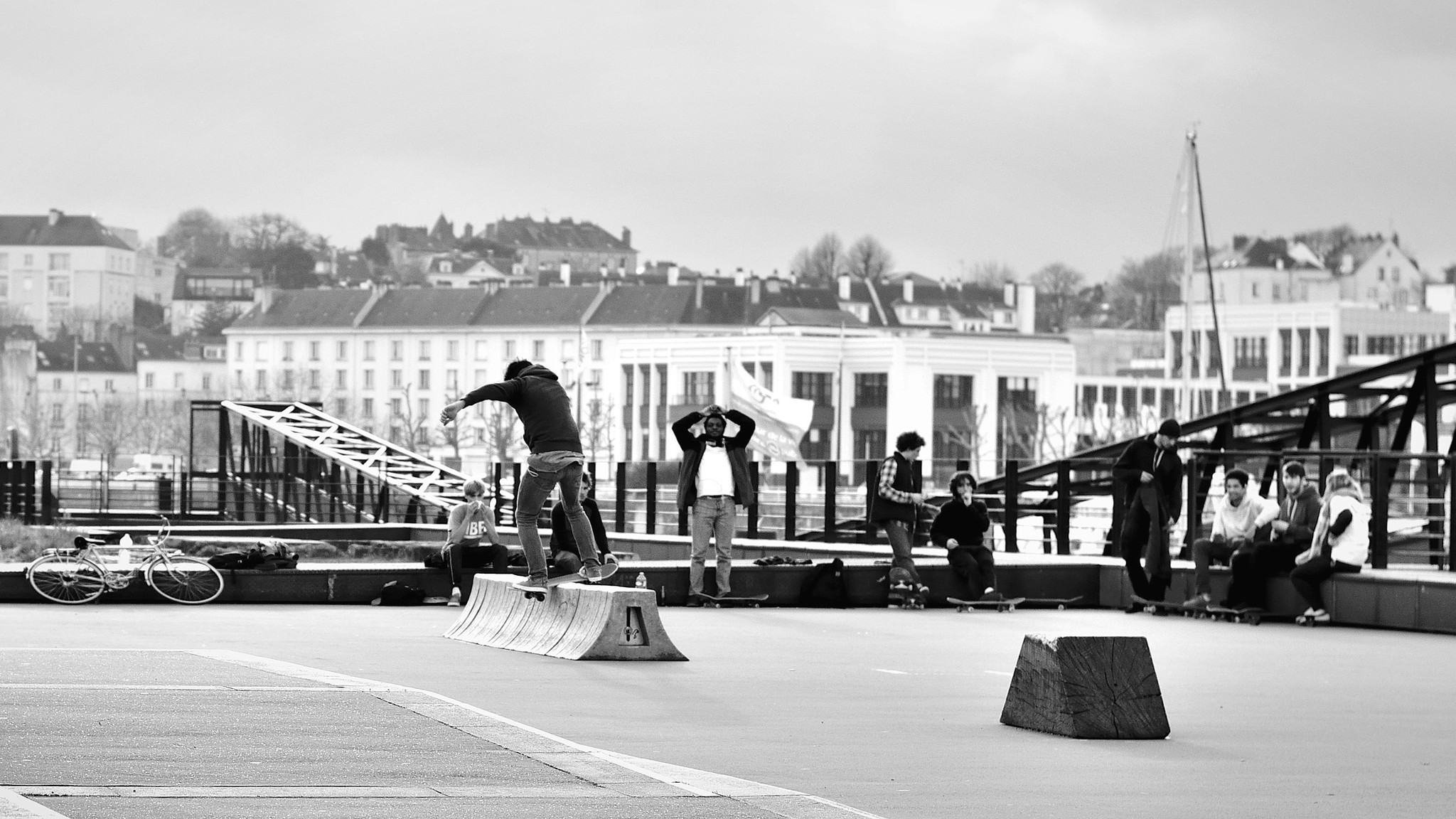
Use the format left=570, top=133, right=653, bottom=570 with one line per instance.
left=564, top=377, right=596, bottom=435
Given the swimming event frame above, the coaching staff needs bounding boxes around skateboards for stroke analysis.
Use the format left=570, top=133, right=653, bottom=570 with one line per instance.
left=999, top=595, right=1084, bottom=611
left=888, top=567, right=927, bottom=610
left=1130, top=593, right=1264, bottom=625
left=694, top=592, right=769, bottom=608
left=946, top=596, right=1026, bottom=613
left=512, top=563, right=618, bottom=602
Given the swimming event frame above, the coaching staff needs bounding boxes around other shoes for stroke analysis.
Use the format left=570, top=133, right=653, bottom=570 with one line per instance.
left=1126, top=601, right=1146, bottom=613
left=979, top=591, right=1003, bottom=601
left=920, top=586, right=930, bottom=601
left=1221, top=599, right=1243, bottom=610
left=686, top=595, right=700, bottom=607
left=512, top=577, right=549, bottom=594
left=1304, top=607, right=1325, bottom=616
left=579, top=565, right=602, bottom=582
left=447, top=593, right=460, bottom=607
left=1296, top=614, right=1330, bottom=624
left=720, top=593, right=733, bottom=608
left=1183, top=593, right=1212, bottom=608
left=1138, top=591, right=1165, bottom=602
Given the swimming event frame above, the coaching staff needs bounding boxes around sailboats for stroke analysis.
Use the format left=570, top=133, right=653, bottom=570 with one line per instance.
left=984, top=131, right=1430, bottom=562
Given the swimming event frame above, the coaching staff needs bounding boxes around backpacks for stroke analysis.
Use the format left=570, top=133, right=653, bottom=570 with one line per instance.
left=797, top=558, right=850, bottom=610
left=206, top=552, right=249, bottom=571
left=380, top=581, right=427, bottom=604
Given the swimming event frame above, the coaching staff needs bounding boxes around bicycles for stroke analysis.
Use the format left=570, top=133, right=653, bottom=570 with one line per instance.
left=24, top=514, right=224, bottom=606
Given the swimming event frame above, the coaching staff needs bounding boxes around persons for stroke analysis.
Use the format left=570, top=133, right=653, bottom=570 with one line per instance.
left=1112, top=419, right=1183, bottom=615
left=1181, top=469, right=1281, bottom=607
left=1288, top=469, right=1372, bottom=624
left=439, top=359, right=603, bottom=593
left=930, top=472, right=1004, bottom=606
left=870, top=432, right=943, bottom=609
left=670, top=404, right=756, bottom=608
left=550, top=470, right=620, bottom=586
left=1220, top=459, right=1321, bottom=612
left=440, top=477, right=510, bottom=607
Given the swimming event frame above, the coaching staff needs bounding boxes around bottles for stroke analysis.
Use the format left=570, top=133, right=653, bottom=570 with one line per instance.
left=635, top=571, right=647, bottom=589
left=119, top=534, right=132, bottom=568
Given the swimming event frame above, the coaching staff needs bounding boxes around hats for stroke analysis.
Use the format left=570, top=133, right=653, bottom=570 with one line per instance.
left=1158, top=419, right=1181, bottom=437
